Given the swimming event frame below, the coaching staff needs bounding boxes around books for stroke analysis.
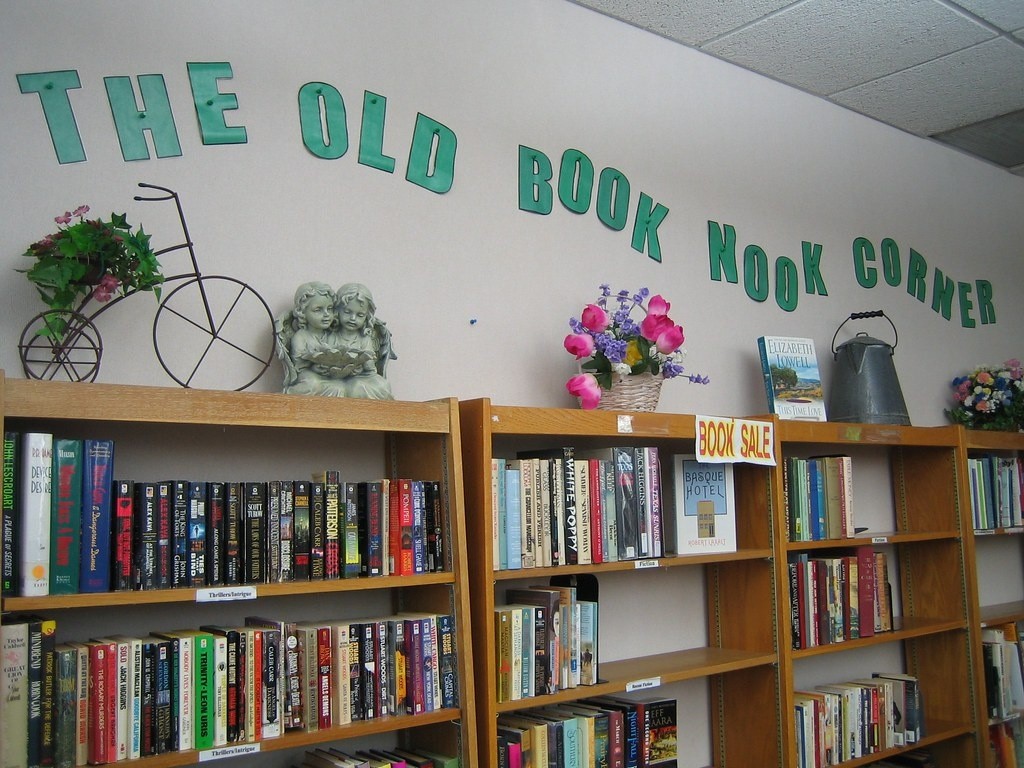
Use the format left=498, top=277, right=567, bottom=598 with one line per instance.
left=981, top=622, right=1024, bottom=768
left=757, top=336, right=827, bottom=422
left=967, top=455, right=1024, bottom=530
left=671, top=454, right=736, bottom=555
left=782, top=454, right=920, bottom=768
left=0, top=431, right=461, bottom=768
left=492, top=446, right=677, bottom=768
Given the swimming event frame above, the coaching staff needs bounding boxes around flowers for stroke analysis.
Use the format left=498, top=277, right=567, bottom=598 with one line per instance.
left=943, top=358, right=1024, bottom=433
left=11, top=204, right=166, bottom=341
left=564, top=282, right=710, bottom=411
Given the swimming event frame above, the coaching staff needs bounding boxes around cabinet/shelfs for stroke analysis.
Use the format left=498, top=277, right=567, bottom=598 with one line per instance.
left=0, top=370, right=1024, bottom=768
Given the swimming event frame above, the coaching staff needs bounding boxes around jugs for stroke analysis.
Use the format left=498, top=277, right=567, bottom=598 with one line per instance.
left=828, top=310, right=912, bottom=426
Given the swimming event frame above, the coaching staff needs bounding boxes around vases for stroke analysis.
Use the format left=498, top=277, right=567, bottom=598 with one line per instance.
left=53, top=243, right=110, bottom=285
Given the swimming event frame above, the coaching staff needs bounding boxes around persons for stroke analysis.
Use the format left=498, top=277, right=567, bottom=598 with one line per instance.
left=290, top=281, right=390, bottom=400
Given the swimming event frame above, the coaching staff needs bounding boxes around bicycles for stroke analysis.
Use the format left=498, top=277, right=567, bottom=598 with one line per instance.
left=16, top=178, right=280, bottom=394
